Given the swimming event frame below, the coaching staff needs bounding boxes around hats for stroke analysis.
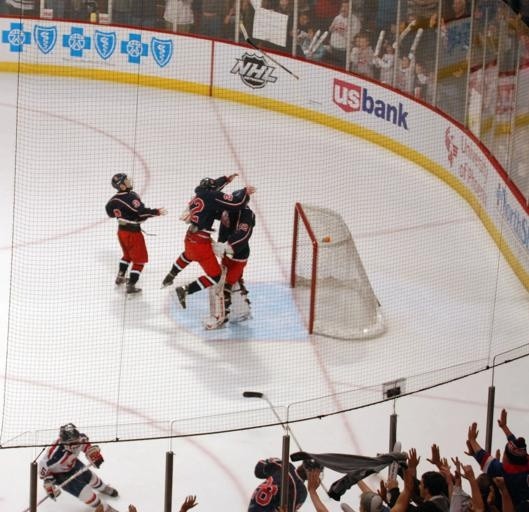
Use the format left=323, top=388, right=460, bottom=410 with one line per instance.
left=506, top=438, right=528, bottom=458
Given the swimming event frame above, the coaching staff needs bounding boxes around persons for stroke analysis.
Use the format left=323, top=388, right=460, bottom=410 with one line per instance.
left=204, top=189, right=257, bottom=330
left=1, top=1, right=529, bottom=101
left=37, top=422, right=119, bottom=507
left=95, top=504, right=137, bottom=512
left=104, top=173, right=169, bottom=294
left=246, top=408, right=529, bottom=512
left=178, top=494, right=199, bottom=512
left=159, top=172, right=259, bottom=309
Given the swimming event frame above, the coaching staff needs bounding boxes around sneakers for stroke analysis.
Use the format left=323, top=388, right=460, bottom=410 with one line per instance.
left=163, top=279, right=173, bottom=285
left=176, top=287, right=188, bottom=307
left=116, top=276, right=141, bottom=293
left=104, top=487, right=117, bottom=497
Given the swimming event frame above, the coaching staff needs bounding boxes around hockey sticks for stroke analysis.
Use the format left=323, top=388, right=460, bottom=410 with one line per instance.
left=243, top=391, right=328, bottom=495
left=140, top=228, right=157, bottom=236
left=238, top=23, right=299, bottom=81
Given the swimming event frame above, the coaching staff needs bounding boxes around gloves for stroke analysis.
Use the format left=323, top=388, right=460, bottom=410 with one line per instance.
left=45, top=482, right=60, bottom=499
left=211, top=242, right=234, bottom=256
left=85, top=445, right=104, bottom=468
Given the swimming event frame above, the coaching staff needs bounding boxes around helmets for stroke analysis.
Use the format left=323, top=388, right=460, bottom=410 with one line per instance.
left=60, top=424, right=81, bottom=452
left=112, top=173, right=132, bottom=191
left=233, top=189, right=249, bottom=207
left=200, top=178, right=217, bottom=192
